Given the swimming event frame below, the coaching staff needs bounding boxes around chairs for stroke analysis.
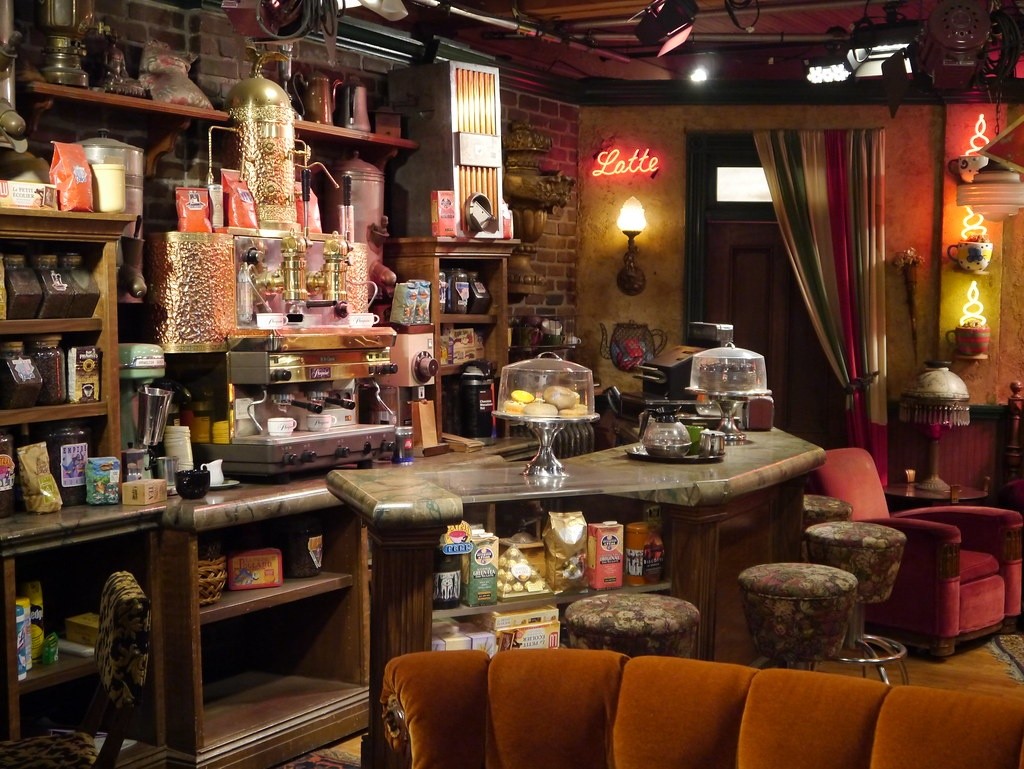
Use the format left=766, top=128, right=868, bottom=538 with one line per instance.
left=0, top=569, right=151, bottom=769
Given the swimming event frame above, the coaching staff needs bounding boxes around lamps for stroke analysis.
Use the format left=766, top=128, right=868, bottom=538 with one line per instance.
left=896, top=360, right=970, bottom=492
left=632, top=0, right=695, bottom=60
left=806, top=0, right=921, bottom=86
left=617, top=196, right=648, bottom=296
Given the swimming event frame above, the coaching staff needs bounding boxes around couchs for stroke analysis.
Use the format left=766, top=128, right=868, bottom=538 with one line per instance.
left=376, top=648, right=1024, bottom=768
left=802, top=447, right=1023, bottom=658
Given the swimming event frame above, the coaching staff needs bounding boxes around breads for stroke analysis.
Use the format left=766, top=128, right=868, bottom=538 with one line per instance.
left=502, top=385, right=589, bottom=416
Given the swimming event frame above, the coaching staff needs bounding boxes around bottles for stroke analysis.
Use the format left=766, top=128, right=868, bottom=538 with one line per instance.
left=0, top=250, right=100, bottom=520
left=439, top=268, right=490, bottom=314
left=395, top=426, right=414, bottom=458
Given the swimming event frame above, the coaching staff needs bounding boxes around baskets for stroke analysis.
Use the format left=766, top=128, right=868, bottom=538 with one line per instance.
left=198, top=555, right=227, bottom=604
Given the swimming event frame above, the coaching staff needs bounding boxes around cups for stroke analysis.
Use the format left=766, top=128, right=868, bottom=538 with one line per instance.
left=205, top=184, right=224, bottom=228
left=945, top=326, right=990, bottom=357
left=508, top=318, right=582, bottom=352
left=347, top=312, right=380, bottom=328
left=307, top=414, right=338, bottom=432
left=256, top=312, right=289, bottom=330
left=700, top=429, right=727, bottom=455
left=948, top=155, right=988, bottom=184
left=157, top=455, right=179, bottom=496
left=685, top=424, right=703, bottom=455
left=947, top=241, right=993, bottom=271
left=173, top=469, right=210, bottom=499
left=268, top=417, right=297, bottom=437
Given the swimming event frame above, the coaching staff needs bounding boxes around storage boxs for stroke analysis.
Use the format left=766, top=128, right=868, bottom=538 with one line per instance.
left=226, top=546, right=285, bottom=589
left=430, top=604, right=561, bottom=654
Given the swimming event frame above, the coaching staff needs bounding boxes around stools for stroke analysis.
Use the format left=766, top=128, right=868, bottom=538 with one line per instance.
left=566, top=593, right=700, bottom=661
left=804, top=519, right=911, bottom=686
left=739, top=563, right=858, bottom=672
left=803, top=491, right=854, bottom=528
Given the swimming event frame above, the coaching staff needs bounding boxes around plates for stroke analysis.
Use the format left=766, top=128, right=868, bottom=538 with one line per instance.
left=209, top=480, right=241, bottom=492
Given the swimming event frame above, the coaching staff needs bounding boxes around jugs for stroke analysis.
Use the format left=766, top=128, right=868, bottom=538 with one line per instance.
left=637, top=403, right=693, bottom=460
left=289, top=69, right=342, bottom=128
left=201, top=458, right=224, bottom=486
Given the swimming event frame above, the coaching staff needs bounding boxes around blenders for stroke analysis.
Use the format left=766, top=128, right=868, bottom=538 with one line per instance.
left=118, top=342, right=167, bottom=480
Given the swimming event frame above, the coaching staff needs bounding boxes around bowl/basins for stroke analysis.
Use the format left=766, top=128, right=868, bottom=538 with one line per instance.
left=541, top=320, right=562, bottom=335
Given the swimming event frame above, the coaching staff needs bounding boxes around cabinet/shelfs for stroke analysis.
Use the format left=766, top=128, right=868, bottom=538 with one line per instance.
left=0, top=62, right=420, bottom=769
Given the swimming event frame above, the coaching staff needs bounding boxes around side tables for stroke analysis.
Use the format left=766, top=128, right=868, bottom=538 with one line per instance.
left=881, top=479, right=988, bottom=505
